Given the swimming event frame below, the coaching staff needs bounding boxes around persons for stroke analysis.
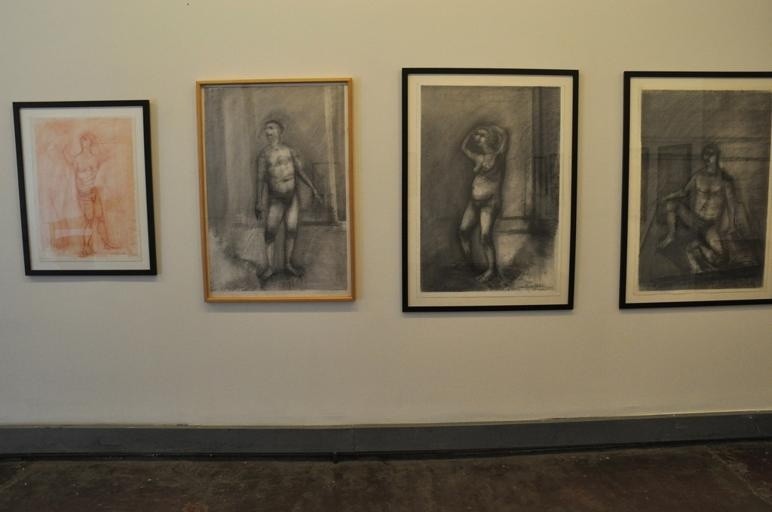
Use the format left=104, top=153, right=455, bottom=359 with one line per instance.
left=63, top=132, right=121, bottom=258
left=255, top=119, right=324, bottom=280
left=658, top=141, right=737, bottom=274
left=457, top=123, right=509, bottom=283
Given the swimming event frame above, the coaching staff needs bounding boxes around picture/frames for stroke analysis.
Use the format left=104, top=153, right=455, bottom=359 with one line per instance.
left=194, top=77, right=357, bottom=304
left=12, top=100, right=158, bottom=277
left=400, top=66, right=579, bottom=313
left=618, top=70, right=772, bottom=309
